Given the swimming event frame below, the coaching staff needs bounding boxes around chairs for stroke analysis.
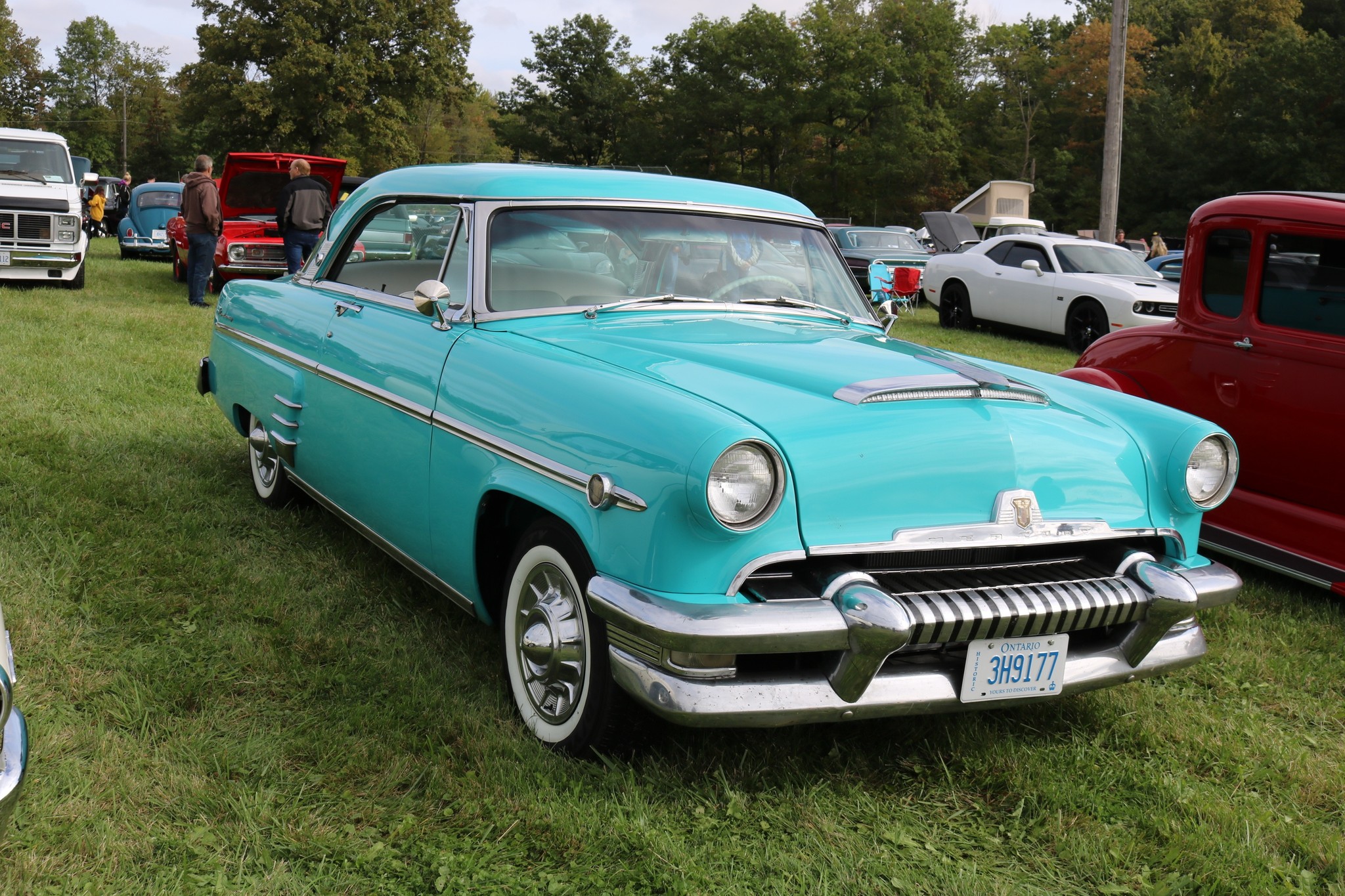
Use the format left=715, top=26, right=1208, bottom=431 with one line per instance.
left=874, top=266, right=921, bottom=317
left=868, top=264, right=909, bottom=313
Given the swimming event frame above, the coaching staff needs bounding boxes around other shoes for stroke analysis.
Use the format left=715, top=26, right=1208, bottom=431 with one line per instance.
left=105, top=233, right=112, bottom=238
left=190, top=301, right=211, bottom=309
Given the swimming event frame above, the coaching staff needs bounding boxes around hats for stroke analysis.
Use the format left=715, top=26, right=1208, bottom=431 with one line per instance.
left=1150, top=232, right=1161, bottom=236
left=1115, top=229, right=1125, bottom=239
left=148, top=173, right=158, bottom=179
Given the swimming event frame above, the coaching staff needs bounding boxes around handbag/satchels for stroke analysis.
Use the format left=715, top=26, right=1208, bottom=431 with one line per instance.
left=114, top=184, right=127, bottom=211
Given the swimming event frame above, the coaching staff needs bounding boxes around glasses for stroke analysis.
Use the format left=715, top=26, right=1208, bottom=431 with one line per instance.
left=288, top=166, right=296, bottom=171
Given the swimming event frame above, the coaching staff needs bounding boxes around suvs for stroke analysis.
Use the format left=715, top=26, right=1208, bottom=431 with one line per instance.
left=0, top=127, right=91, bottom=291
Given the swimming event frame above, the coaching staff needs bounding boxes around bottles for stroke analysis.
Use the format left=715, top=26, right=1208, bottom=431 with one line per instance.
left=879, top=292, right=886, bottom=303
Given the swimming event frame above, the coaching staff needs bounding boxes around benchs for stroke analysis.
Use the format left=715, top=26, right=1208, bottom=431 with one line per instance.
left=336, top=259, right=629, bottom=304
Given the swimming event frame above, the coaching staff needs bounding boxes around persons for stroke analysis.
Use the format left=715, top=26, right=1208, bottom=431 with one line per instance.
left=89, top=186, right=112, bottom=239
left=1113, top=228, right=1131, bottom=251
left=179, top=154, right=223, bottom=308
left=276, top=159, right=334, bottom=275
left=1144, top=231, right=1168, bottom=262
left=146, top=174, right=156, bottom=183
left=117, top=172, right=132, bottom=219
left=424, top=207, right=435, bottom=223
left=724, top=228, right=759, bottom=304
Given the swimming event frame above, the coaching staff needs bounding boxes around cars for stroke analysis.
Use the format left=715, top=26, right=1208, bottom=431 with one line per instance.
left=118, top=181, right=187, bottom=259
left=70, top=155, right=134, bottom=240
left=165, top=152, right=1187, bottom=358
left=1054, top=189, right=1345, bottom=597
left=192, top=162, right=1242, bottom=759
